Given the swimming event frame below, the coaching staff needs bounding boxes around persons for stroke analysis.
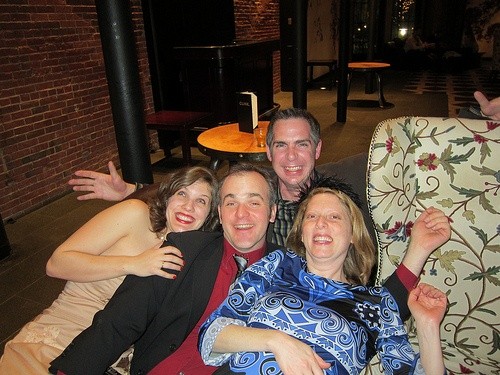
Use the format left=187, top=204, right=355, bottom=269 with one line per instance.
left=403, top=28, right=482, bottom=74
left=67, top=107, right=362, bottom=248
left=0, top=163, right=221, bottom=375
left=457, top=90, right=500, bottom=121
left=46, top=162, right=453, bottom=375
left=196, top=186, right=448, bottom=375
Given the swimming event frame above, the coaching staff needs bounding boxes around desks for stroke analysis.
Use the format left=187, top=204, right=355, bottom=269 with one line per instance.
left=195, top=121, right=269, bottom=173
left=307, top=59, right=337, bottom=90
left=347, top=62, right=391, bottom=109
left=145, top=110, right=212, bottom=168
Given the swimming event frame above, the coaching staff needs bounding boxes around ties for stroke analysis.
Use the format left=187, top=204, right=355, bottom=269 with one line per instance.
left=232, top=253, right=248, bottom=281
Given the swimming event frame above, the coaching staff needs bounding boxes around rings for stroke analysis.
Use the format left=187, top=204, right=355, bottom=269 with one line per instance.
left=162, top=262, right=164, bottom=268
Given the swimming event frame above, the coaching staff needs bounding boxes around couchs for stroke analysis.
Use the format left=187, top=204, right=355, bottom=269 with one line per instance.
left=123, top=117, right=500, bottom=375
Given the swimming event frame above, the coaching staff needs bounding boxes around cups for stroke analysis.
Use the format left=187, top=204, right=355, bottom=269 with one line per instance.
left=253, top=128, right=267, bottom=147
